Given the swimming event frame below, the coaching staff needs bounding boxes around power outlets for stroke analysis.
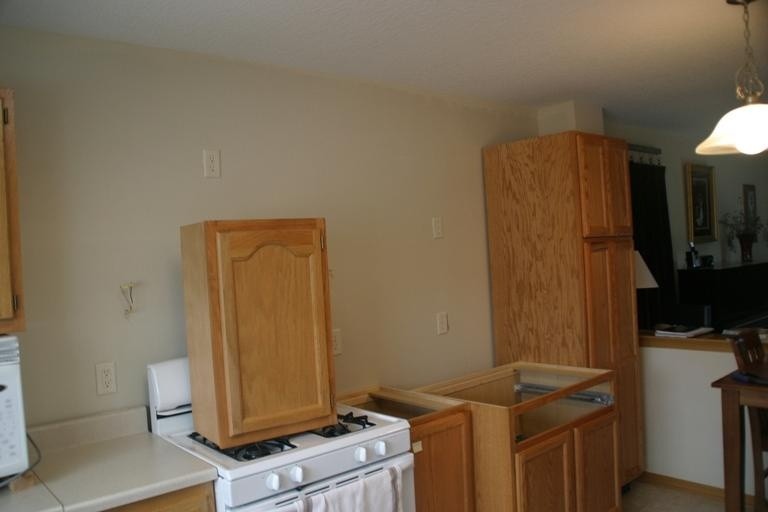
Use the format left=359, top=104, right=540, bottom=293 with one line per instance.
left=432, top=216, right=445, bottom=239
left=95, top=361, right=115, bottom=396
left=437, top=310, right=449, bottom=335
left=332, top=328, right=343, bottom=354
left=204, top=148, right=221, bottom=176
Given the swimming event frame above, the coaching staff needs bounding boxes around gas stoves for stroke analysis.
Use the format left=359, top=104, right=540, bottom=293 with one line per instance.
left=168, top=392, right=410, bottom=508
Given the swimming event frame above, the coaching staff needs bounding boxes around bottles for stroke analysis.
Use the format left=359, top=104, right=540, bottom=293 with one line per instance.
left=689, top=242, right=700, bottom=268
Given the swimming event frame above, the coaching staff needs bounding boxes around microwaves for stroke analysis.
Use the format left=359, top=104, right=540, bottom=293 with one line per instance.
left=1, top=334, right=33, bottom=483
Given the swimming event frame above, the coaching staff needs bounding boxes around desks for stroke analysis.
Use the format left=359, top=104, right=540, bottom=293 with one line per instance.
left=714, top=261, right=767, bottom=333
left=712, top=353, right=768, bottom=512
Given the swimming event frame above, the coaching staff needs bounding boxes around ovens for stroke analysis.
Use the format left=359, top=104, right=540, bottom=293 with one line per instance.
left=215, top=451, right=416, bottom=512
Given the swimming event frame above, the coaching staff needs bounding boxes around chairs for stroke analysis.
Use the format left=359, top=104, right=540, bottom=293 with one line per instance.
left=729, top=330, right=768, bottom=512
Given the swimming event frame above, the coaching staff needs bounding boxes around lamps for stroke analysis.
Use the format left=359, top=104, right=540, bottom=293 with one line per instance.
left=696, top=0, right=768, bottom=155
left=636, top=250, right=660, bottom=289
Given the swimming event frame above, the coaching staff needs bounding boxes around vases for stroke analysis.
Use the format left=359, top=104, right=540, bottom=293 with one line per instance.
left=737, top=233, right=754, bottom=263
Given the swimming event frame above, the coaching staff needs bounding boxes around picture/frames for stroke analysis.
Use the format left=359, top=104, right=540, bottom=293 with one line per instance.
left=742, top=184, right=758, bottom=241
left=686, top=162, right=719, bottom=244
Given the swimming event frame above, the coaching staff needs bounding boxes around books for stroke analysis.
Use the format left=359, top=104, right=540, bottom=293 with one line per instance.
left=654, top=323, right=715, bottom=338
left=721, top=327, right=768, bottom=336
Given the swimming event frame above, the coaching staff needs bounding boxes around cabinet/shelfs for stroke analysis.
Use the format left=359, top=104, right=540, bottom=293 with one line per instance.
left=340, top=385, right=476, bottom=510
left=414, top=359, right=624, bottom=511
left=0, top=88, right=28, bottom=335
left=178, top=217, right=339, bottom=451
left=98, top=434, right=219, bottom=512
left=482, top=131, right=645, bottom=493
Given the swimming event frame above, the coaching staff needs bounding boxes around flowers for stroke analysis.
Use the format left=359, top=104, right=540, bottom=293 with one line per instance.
left=718, top=194, right=767, bottom=250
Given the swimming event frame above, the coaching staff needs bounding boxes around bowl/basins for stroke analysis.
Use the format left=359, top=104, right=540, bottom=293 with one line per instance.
left=698, top=255, right=714, bottom=267
